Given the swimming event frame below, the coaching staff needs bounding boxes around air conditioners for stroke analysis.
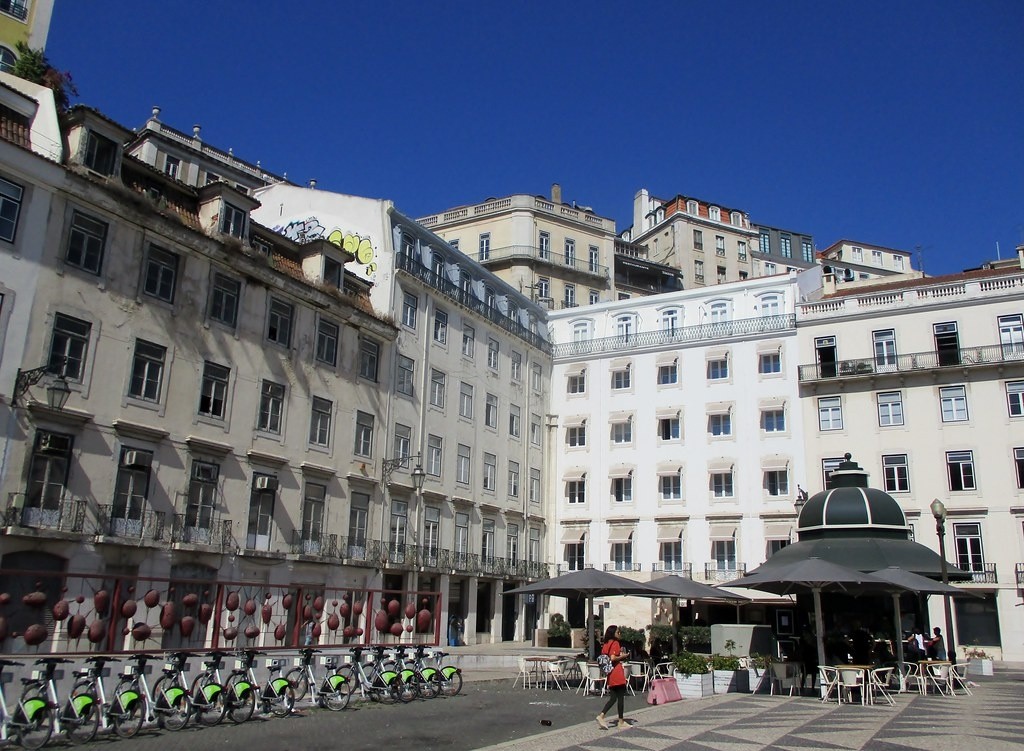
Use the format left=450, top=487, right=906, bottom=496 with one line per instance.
left=40, top=433, right=73, bottom=452
left=258, top=477, right=279, bottom=489
left=125, top=450, right=155, bottom=468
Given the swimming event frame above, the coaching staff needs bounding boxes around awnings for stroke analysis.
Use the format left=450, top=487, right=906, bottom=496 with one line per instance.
left=564, top=343, right=781, bottom=377
left=560, top=525, right=793, bottom=544
left=562, top=459, right=788, bottom=482
left=616, top=256, right=683, bottom=278
left=562, top=400, right=786, bottom=428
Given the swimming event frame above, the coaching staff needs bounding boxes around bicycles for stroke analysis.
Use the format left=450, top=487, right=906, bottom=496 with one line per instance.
left=399, top=644, right=442, bottom=699
left=406, top=645, right=463, bottom=697
left=224, top=650, right=295, bottom=717
left=337, top=646, right=403, bottom=705
left=151, top=651, right=228, bottom=726
left=71, top=657, right=147, bottom=738
left=286, top=648, right=351, bottom=711
left=116, top=654, right=193, bottom=732
left=20, top=657, right=103, bottom=744
left=357, top=645, right=420, bottom=703
left=0, top=659, right=60, bottom=751
left=190, top=650, right=255, bottom=724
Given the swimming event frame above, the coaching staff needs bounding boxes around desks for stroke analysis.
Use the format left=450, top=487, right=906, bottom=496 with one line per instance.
left=834, top=665, right=872, bottom=703
left=524, top=657, right=554, bottom=691
left=917, top=659, right=950, bottom=693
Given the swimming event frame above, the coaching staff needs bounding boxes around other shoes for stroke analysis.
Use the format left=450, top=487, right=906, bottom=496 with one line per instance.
left=618, top=721, right=633, bottom=729
left=596, top=715, right=608, bottom=730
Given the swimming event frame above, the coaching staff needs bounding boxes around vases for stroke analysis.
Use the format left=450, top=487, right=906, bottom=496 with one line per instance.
left=968, top=655, right=996, bottom=676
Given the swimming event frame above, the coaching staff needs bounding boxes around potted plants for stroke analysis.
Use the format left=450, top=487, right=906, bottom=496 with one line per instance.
left=748, top=652, right=776, bottom=690
left=647, top=625, right=673, bottom=662
left=546, top=613, right=571, bottom=649
left=711, top=640, right=750, bottom=693
left=675, top=655, right=715, bottom=699
left=618, top=626, right=646, bottom=657
left=680, top=625, right=711, bottom=654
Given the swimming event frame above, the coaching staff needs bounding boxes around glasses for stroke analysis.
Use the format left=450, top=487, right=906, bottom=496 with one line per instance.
left=614, top=631, right=621, bottom=635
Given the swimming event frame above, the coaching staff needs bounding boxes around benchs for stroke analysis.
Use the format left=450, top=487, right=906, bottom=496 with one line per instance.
left=839, top=364, right=873, bottom=375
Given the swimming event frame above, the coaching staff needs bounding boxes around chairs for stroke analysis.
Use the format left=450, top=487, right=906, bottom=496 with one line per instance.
left=770, top=658, right=973, bottom=706
left=512, top=655, right=677, bottom=695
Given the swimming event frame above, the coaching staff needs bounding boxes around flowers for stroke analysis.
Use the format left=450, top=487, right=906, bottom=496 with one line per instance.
left=962, top=641, right=991, bottom=661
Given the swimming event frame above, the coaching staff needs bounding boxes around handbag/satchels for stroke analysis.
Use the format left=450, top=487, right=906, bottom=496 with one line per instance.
left=908, top=634, right=918, bottom=653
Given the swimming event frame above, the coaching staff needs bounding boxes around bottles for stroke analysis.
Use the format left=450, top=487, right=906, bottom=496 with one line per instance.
left=539, top=720, right=552, bottom=726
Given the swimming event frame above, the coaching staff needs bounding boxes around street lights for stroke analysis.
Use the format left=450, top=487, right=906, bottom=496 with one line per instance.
left=930, top=500, right=962, bottom=690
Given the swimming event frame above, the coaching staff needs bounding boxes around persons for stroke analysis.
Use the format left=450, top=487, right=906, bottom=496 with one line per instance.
left=301, top=616, right=317, bottom=652
left=596, top=626, right=633, bottom=729
left=620, top=637, right=672, bottom=660
left=594, top=635, right=601, bottom=658
left=908, top=626, right=946, bottom=660
left=799, top=619, right=893, bottom=691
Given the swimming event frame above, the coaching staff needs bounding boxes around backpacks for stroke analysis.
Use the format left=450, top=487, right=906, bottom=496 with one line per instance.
left=597, top=642, right=616, bottom=675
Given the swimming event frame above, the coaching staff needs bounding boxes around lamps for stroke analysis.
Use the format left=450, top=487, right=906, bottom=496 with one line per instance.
left=12, top=356, right=72, bottom=415
left=793, top=485, right=808, bottom=516
left=380, top=452, right=426, bottom=489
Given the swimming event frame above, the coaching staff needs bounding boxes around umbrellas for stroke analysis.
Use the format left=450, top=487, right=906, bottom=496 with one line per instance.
left=632, top=572, right=751, bottom=655
left=498, top=562, right=681, bottom=660
left=868, top=566, right=985, bottom=675
left=713, top=555, right=914, bottom=698
